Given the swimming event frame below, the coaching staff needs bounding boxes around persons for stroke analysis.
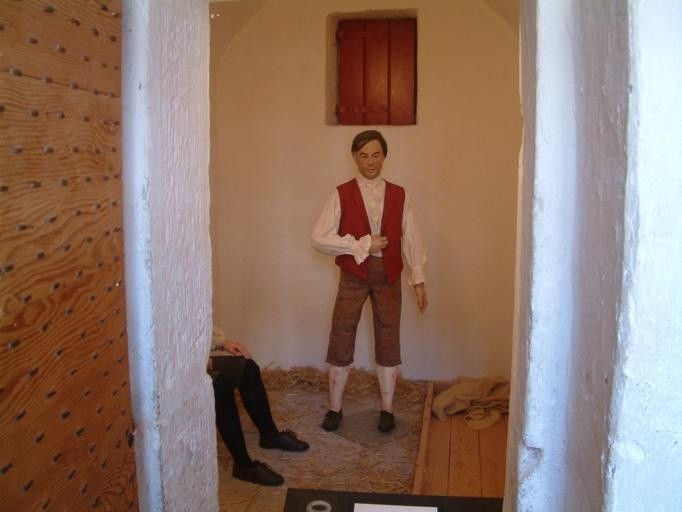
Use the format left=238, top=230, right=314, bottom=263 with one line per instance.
left=207, top=325, right=309, bottom=486
left=311, top=126, right=427, bottom=431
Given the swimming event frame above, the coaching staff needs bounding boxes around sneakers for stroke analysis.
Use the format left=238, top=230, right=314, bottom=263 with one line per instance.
left=379, top=411, right=395, bottom=432
left=323, top=408, right=342, bottom=432
left=234, top=461, right=283, bottom=486
left=262, top=429, right=308, bottom=451
